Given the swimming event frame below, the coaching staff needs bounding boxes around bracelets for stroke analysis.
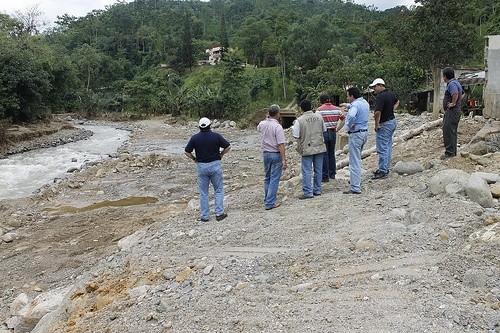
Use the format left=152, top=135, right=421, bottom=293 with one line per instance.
left=193, top=157, right=196, bottom=160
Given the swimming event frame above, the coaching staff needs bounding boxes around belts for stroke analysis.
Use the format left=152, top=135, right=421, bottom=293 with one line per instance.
left=382, top=116, right=395, bottom=122
left=348, top=129, right=368, bottom=134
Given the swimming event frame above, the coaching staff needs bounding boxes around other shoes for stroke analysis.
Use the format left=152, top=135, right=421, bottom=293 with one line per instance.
left=216, top=213, right=227, bottom=221
left=299, top=194, right=306, bottom=199
left=322, top=178, right=328, bottom=181
left=343, top=190, right=361, bottom=194
left=201, top=218, right=208, bottom=222
left=371, top=171, right=385, bottom=180
left=265, top=203, right=280, bottom=210
left=373, top=170, right=388, bottom=178
left=440, top=153, right=455, bottom=159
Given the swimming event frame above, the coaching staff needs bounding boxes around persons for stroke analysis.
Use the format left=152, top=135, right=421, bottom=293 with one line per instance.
left=315, top=93, right=345, bottom=182
left=257, top=104, right=287, bottom=210
left=184, top=117, right=231, bottom=222
left=292, top=99, right=327, bottom=199
left=339, top=87, right=370, bottom=194
left=369, top=78, right=400, bottom=179
left=440, top=67, right=462, bottom=160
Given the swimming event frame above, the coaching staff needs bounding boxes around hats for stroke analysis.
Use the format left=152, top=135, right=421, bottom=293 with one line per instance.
left=369, top=78, right=385, bottom=87
left=199, top=117, right=212, bottom=128
